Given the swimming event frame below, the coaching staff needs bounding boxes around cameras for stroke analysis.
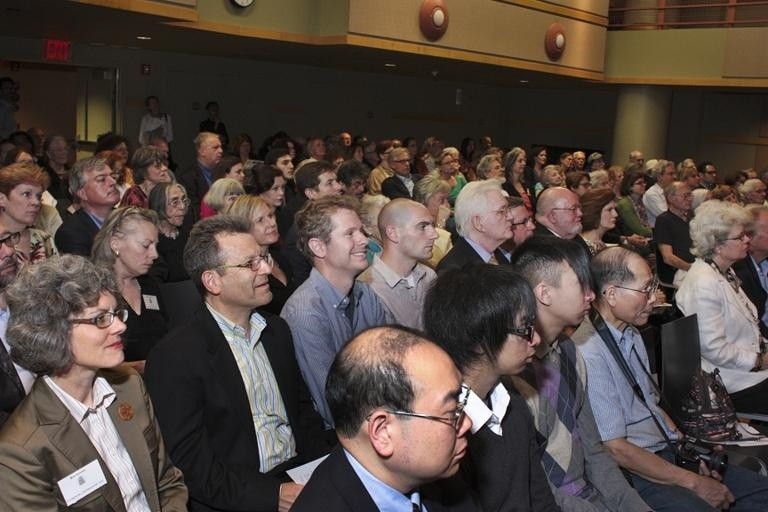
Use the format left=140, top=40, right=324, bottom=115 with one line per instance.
left=674, top=434, right=729, bottom=477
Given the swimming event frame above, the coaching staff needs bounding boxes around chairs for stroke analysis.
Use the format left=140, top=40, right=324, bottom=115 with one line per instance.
left=658, top=311, right=767, bottom=478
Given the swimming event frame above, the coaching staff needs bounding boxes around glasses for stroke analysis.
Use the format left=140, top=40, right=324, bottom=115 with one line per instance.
left=551, top=205, right=581, bottom=212
left=391, top=159, right=409, bottom=163
left=167, top=198, right=190, bottom=207
left=506, top=323, right=535, bottom=343
left=723, top=233, right=746, bottom=242
left=512, top=215, right=533, bottom=226
left=603, top=282, right=658, bottom=294
left=68, top=305, right=129, bottom=328
left=367, top=384, right=471, bottom=431
left=218, top=251, right=270, bottom=271
left=0, top=232, right=20, bottom=250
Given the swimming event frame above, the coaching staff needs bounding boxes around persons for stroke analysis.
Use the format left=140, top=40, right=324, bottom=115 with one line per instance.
left=199, top=100, right=229, bottom=148
left=0, top=77, right=21, bottom=139
left=0, top=128, right=767, bottom=512
left=137, top=95, right=174, bottom=168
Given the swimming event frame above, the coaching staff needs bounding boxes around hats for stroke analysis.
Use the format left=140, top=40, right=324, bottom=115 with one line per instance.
left=587, top=151, right=602, bottom=163
